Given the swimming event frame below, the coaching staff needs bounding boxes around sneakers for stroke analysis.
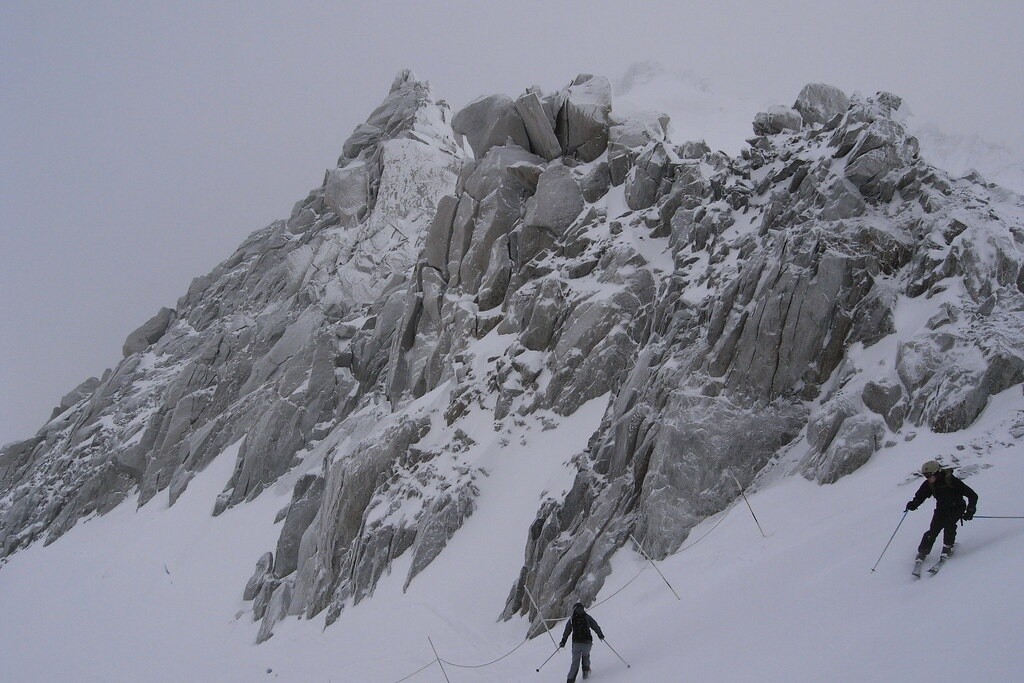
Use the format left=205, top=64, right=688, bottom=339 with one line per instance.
left=567, top=679, right=574, bottom=683
left=582, top=666, right=589, bottom=671
left=915, top=552, right=926, bottom=563
left=941, top=545, right=952, bottom=558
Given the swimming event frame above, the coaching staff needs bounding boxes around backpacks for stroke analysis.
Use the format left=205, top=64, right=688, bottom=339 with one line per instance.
left=571, top=614, right=591, bottom=641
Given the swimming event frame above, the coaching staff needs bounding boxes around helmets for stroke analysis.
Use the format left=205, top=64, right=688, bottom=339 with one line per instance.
left=573, top=603, right=583, bottom=612
left=922, top=461, right=939, bottom=475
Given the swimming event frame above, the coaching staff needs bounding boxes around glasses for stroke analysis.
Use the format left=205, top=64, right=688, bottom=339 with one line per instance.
left=923, top=472, right=935, bottom=478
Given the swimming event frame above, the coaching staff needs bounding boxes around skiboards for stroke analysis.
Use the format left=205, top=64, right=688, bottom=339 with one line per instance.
left=575, top=668, right=593, bottom=683
left=911, top=541, right=959, bottom=577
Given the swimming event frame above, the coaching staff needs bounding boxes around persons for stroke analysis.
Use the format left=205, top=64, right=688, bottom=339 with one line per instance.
left=904, top=460, right=979, bottom=578
left=560, top=602, right=606, bottom=683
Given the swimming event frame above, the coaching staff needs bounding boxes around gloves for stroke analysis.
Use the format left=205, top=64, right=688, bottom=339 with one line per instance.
left=963, top=513, right=973, bottom=521
left=600, top=635, right=605, bottom=641
left=559, top=642, right=565, bottom=647
left=907, top=501, right=916, bottom=511
